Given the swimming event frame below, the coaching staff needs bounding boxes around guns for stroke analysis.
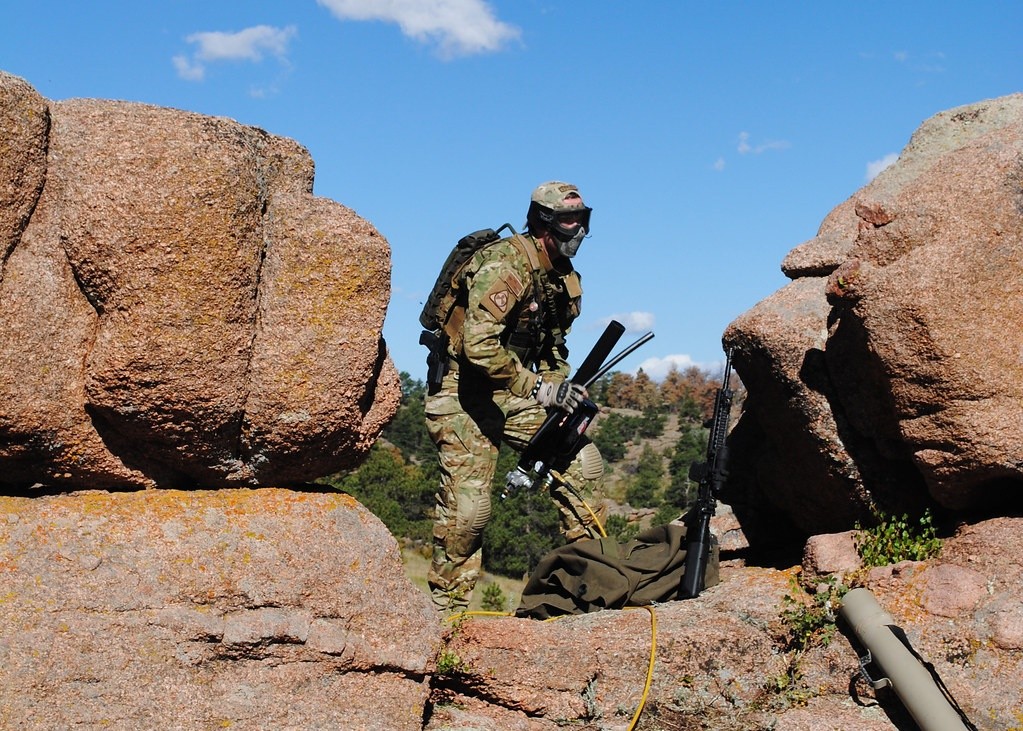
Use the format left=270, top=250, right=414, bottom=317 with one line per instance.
left=501, top=319, right=655, bottom=500
left=674, top=347, right=737, bottom=598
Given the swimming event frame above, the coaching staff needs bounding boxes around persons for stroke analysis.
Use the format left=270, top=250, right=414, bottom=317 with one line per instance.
left=423, top=181, right=606, bottom=612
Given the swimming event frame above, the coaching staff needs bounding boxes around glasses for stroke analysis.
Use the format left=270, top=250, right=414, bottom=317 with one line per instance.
left=550, top=208, right=593, bottom=235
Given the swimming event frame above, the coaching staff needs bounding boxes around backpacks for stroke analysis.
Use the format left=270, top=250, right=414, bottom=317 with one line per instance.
left=419, top=227, right=502, bottom=332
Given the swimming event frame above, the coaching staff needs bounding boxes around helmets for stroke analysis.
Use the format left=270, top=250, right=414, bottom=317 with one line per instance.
left=531, top=180, right=593, bottom=259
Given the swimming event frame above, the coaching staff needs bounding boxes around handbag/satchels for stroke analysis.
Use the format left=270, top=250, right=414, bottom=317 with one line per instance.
left=515, top=524, right=721, bottom=622
